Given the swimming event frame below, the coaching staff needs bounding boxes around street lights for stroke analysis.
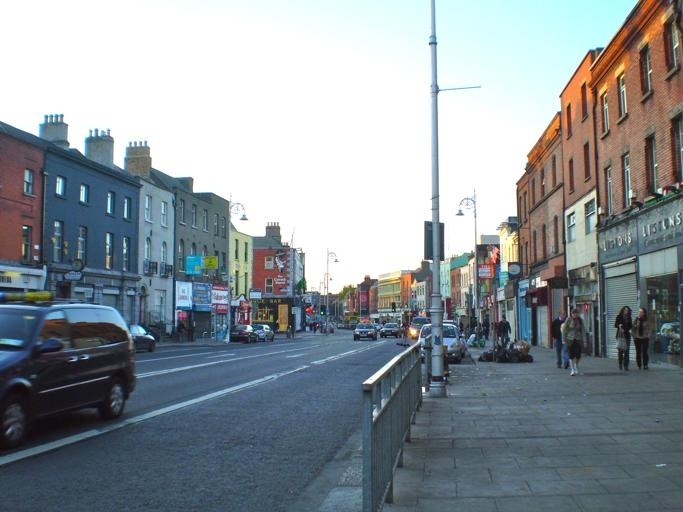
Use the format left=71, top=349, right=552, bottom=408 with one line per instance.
left=453, top=188, right=480, bottom=344
left=225, top=193, right=249, bottom=344
left=310, top=247, right=338, bottom=325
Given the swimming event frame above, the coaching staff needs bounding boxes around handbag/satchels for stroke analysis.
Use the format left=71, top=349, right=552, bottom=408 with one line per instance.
left=616, top=336, right=627, bottom=351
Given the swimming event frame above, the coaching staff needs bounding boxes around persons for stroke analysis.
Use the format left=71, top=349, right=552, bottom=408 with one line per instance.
left=614, top=305, right=634, bottom=372
left=564, top=308, right=588, bottom=376
left=631, top=306, right=650, bottom=371
left=550, top=309, right=572, bottom=369
left=498, top=314, right=511, bottom=350
left=482, top=319, right=491, bottom=342
left=176, top=319, right=187, bottom=344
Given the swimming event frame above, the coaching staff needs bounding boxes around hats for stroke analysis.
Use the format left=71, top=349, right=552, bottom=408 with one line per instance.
left=571, top=309, right=580, bottom=314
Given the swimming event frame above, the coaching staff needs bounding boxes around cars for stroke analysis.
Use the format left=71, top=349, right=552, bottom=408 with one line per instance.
left=125, top=322, right=156, bottom=352
left=318, top=320, right=401, bottom=340
left=654, top=322, right=680, bottom=355
left=228, top=325, right=259, bottom=343
left=254, top=325, right=274, bottom=343
left=407, top=316, right=465, bottom=365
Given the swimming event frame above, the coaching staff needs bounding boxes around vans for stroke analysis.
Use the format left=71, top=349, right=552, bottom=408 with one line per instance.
left=0, top=291, right=138, bottom=449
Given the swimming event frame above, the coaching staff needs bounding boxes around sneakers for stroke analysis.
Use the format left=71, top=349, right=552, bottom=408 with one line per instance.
left=619, top=364, right=648, bottom=370
left=558, top=364, right=578, bottom=376
